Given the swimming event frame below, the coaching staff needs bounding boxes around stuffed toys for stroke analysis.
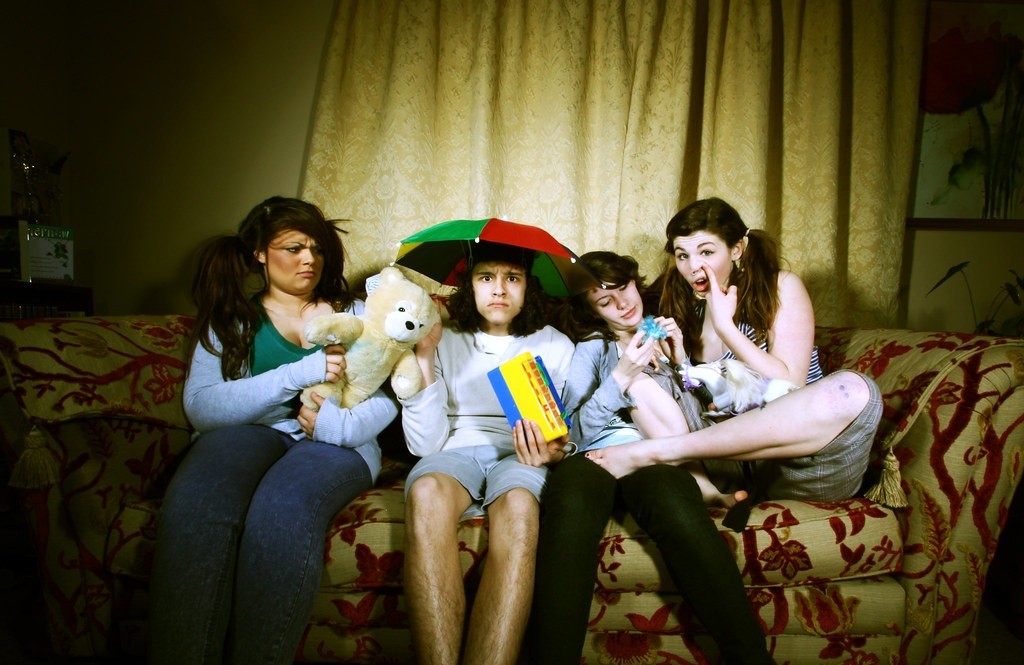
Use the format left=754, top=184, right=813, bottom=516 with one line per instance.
left=300, top=268, right=446, bottom=410
left=680, top=359, right=799, bottom=413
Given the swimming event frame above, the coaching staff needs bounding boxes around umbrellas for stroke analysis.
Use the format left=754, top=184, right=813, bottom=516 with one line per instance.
left=395, top=219, right=603, bottom=298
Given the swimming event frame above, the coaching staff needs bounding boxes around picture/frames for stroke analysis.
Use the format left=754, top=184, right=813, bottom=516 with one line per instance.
left=906, top=0, right=1024, bottom=231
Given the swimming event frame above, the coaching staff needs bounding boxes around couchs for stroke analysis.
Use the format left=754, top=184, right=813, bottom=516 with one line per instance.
left=0, top=312, right=1023, bottom=664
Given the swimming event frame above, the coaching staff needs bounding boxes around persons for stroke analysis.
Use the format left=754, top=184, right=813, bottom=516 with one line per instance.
left=586, top=198, right=885, bottom=502
left=524, top=252, right=772, bottom=664
left=141, top=194, right=400, bottom=665
left=403, top=250, right=587, bottom=665
